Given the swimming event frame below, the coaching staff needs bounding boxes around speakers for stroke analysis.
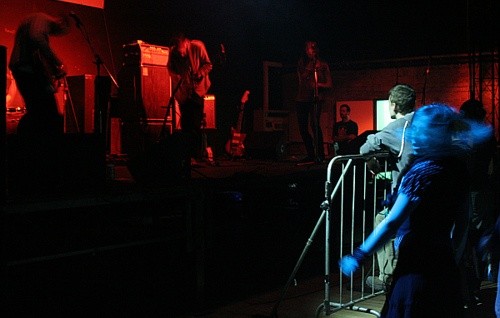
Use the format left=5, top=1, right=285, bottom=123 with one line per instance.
left=4, top=133, right=106, bottom=199
left=129, top=65, right=173, bottom=124
left=123, top=130, right=195, bottom=187
left=67, top=75, right=95, bottom=134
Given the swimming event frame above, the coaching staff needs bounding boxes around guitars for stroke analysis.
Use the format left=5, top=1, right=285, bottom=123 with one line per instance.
left=224, top=89, right=251, bottom=157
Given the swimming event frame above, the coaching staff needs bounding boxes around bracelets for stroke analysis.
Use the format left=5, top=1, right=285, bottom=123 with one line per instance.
left=352, top=248, right=369, bottom=264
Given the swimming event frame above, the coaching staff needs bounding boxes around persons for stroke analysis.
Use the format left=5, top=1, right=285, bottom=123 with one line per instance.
left=171, top=33, right=212, bottom=163
left=456, top=101, right=500, bottom=296
left=339, top=103, right=472, bottom=318
left=332, top=104, right=358, bottom=155
left=291, top=40, right=333, bottom=161
left=8, top=10, right=73, bottom=134
left=365, top=84, right=417, bottom=293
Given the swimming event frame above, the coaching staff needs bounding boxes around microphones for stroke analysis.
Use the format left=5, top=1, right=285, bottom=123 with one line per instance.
left=312, top=57, right=316, bottom=69
left=68, top=11, right=84, bottom=27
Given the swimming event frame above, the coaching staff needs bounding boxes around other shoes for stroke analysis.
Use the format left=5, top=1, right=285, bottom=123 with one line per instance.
left=316, top=157, right=327, bottom=164
left=303, top=156, right=314, bottom=162
left=366, top=276, right=386, bottom=290
